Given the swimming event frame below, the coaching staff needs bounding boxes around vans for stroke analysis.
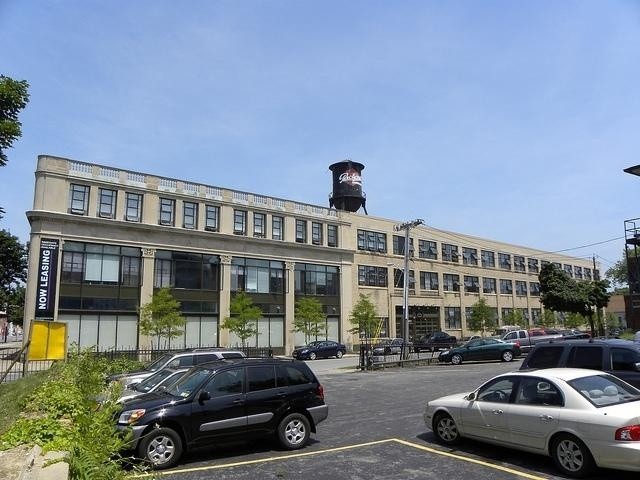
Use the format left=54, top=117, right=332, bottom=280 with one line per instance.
left=518, top=333, right=640, bottom=387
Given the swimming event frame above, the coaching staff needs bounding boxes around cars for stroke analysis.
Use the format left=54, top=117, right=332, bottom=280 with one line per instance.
left=423, top=366, right=640, bottom=477
left=292, top=340, right=346, bottom=361
left=107, top=348, right=247, bottom=404
left=413, top=332, right=458, bottom=352
left=438, top=336, right=522, bottom=365
left=373, top=338, right=414, bottom=356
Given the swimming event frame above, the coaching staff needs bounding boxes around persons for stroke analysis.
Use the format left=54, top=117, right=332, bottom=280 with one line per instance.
left=0, top=320, right=23, bottom=344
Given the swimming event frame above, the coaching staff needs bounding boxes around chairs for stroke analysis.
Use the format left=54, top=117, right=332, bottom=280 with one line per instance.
left=519, top=381, right=540, bottom=404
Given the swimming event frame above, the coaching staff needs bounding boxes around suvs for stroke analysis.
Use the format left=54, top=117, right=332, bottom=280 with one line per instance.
left=111, top=356, right=329, bottom=472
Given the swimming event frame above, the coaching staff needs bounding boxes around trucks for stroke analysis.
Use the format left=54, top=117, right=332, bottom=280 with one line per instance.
left=500, top=330, right=564, bottom=354
